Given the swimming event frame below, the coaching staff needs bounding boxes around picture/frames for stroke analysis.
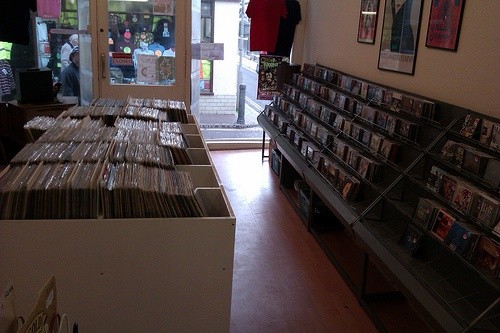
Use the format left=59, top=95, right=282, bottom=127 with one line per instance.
left=425, top=0, right=466, bottom=51
left=357, top=0, right=380, bottom=45
left=377, top=0, right=424, bottom=75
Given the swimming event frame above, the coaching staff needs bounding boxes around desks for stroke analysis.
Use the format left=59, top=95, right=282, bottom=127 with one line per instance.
left=0, top=96, right=77, bottom=162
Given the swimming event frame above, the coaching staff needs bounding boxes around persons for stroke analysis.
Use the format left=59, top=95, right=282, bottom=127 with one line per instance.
left=59, top=46, right=81, bottom=106
left=61, top=33, right=79, bottom=72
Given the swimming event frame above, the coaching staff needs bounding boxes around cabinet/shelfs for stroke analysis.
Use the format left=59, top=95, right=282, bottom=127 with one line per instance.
left=257, top=63, right=500, bottom=333
